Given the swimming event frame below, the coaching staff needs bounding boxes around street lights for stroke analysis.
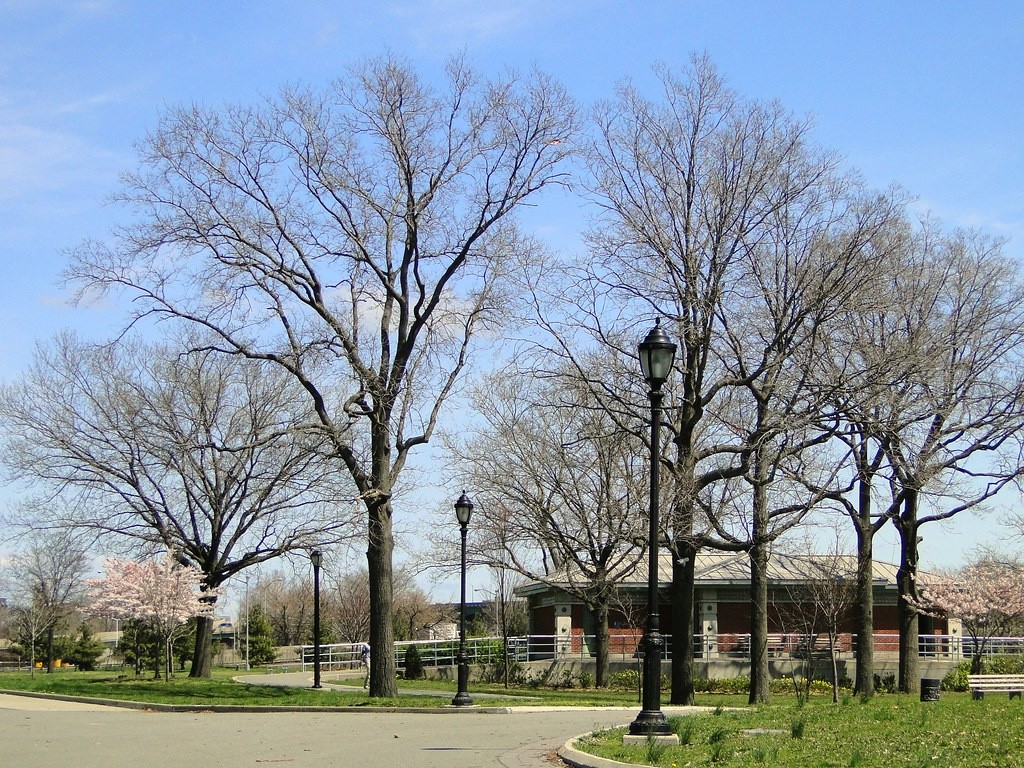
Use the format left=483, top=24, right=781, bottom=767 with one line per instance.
left=303, top=551, right=332, bottom=692
left=263, top=577, right=283, bottom=623
left=620, top=313, right=680, bottom=748
left=450, top=501, right=482, bottom=707
left=475, top=587, right=499, bottom=637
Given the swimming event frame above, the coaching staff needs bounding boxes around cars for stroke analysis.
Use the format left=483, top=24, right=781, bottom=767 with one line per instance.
left=217, top=622, right=233, bottom=631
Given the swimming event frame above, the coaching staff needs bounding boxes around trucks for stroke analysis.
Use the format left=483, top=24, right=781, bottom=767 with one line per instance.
left=417, top=623, right=461, bottom=640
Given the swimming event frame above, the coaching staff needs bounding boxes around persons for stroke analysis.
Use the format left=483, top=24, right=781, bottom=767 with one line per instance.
left=361, top=637, right=370, bottom=689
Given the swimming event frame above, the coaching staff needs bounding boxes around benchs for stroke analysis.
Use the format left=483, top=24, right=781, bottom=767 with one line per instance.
left=967, top=674, right=1024, bottom=700
left=735, top=636, right=784, bottom=657
left=797, top=636, right=844, bottom=659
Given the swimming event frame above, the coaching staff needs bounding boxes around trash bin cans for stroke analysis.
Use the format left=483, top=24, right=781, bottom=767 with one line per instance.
left=921, top=677, right=941, bottom=701
